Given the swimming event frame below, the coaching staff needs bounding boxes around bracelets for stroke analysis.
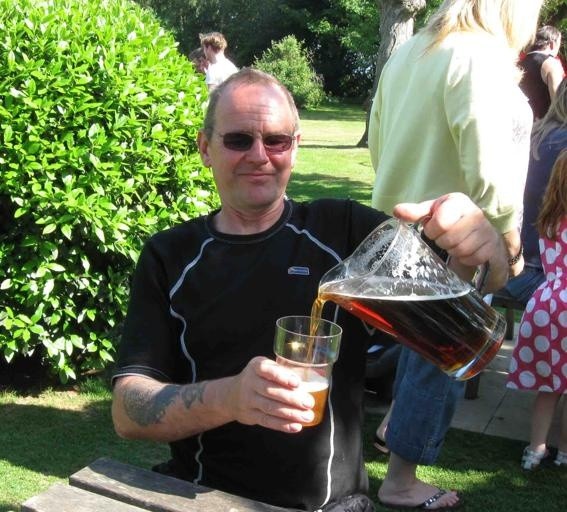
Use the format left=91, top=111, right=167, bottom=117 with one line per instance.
left=508, top=240, right=525, bottom=270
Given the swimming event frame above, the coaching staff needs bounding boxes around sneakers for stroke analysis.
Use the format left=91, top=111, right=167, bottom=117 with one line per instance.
left=520, top=445, right=567, bottom=472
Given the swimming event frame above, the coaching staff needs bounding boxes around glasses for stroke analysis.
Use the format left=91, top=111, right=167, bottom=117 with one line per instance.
left=209, top=127, right=297, bottom=153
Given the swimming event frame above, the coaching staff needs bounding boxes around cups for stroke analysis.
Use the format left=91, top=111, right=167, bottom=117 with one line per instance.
left=273, top=315, right=343, bottom=428
left=315, top=214, right=507, bottom=382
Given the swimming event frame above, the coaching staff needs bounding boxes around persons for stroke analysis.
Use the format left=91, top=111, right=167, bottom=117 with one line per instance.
left=197, top=30, right=241, bottom=92
left=507, top=75, right=566, bottom=309
left=186, top=47, right=210, bottom=83
left=504, top=146, right=566, bottom=472
left=108, top=67, right=515, bottom=511
left=366, top=0, right=547, bottom=512
left=517, top=25, right=566, bottom=120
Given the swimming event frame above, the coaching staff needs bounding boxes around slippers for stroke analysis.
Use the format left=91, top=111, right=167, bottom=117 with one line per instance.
left=378, top=482, right=462, bottom=512
